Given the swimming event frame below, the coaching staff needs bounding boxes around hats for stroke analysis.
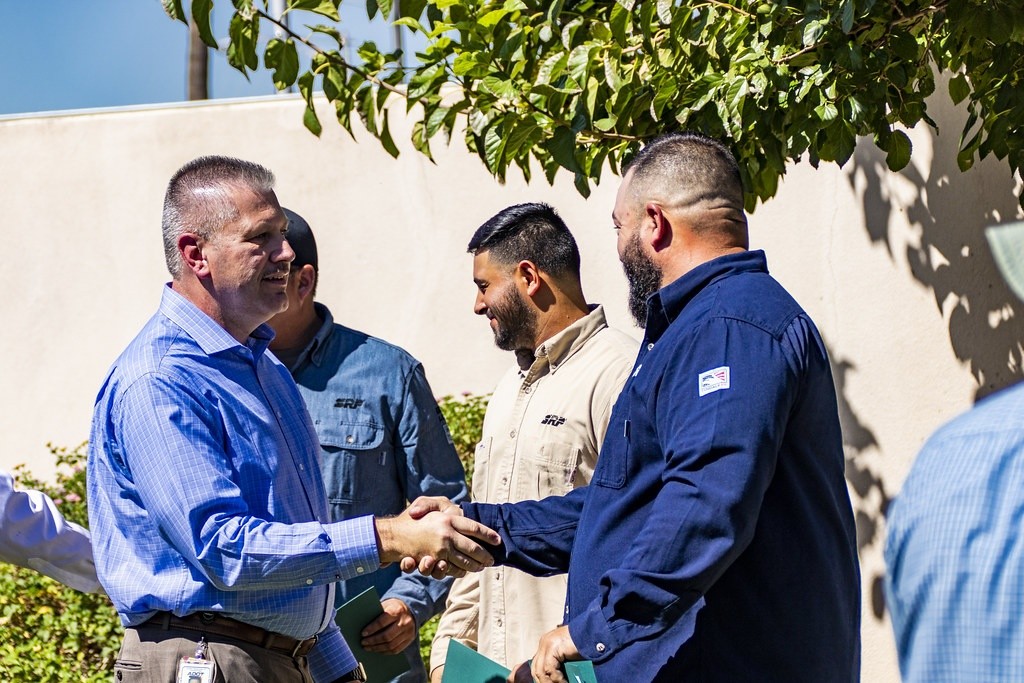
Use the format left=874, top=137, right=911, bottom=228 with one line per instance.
left=282, top=207, right=318, bottom=267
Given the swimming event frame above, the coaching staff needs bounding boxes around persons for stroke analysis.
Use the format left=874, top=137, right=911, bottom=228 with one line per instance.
left=401, top=136, right=864, bottom=683
left=189, top=677, right=202, bottom=683
left=0, top=471, right=108, bottom=595
left=884, top=224, right=1024, bottom=683
left=269, top=206, right=471, bottom=683
left=429, top=201, right=641, bottom=682
left=86, top=156, right=500, bottom=683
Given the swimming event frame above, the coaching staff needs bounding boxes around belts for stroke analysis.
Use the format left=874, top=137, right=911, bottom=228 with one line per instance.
left=150, top=611, right=319, bottom=657
